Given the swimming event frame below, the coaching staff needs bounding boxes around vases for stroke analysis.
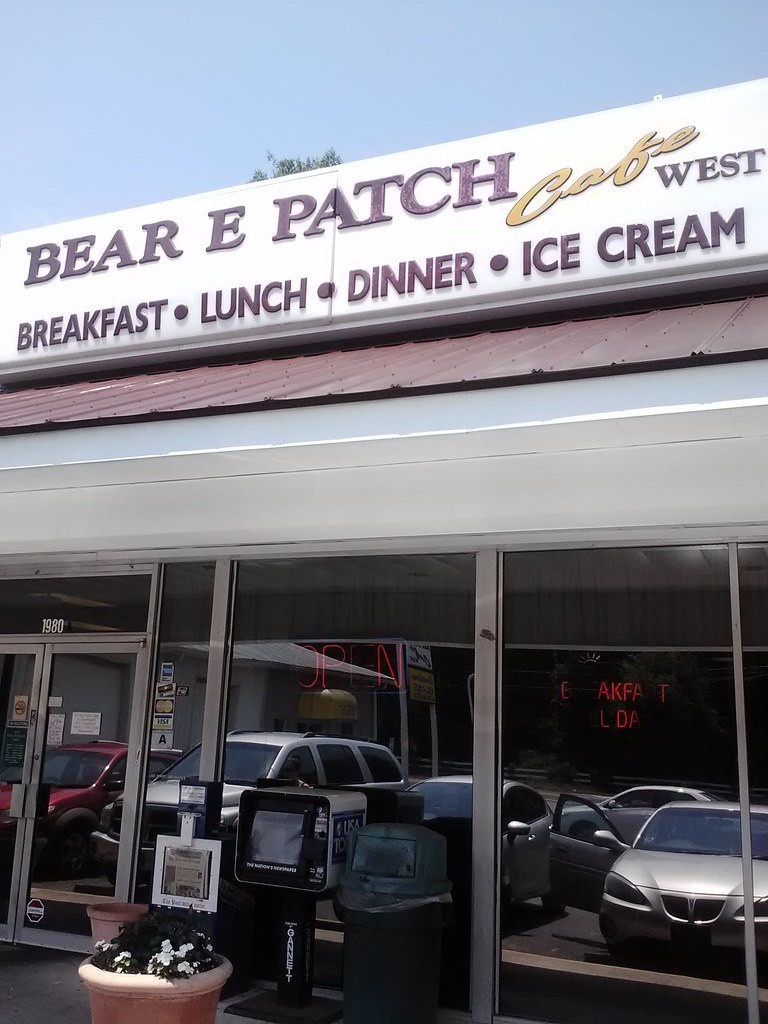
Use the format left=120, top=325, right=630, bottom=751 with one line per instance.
left=78, top=952, right=233, bottom=1024
left=87, top=903, right=150, bottom=949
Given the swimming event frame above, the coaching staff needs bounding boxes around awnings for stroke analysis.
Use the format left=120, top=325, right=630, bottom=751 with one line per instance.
left=298, top=688, right=358, bottom=724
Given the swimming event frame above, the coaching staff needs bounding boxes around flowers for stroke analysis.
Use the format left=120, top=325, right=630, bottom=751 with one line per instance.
left=94, top=905, right=219, bottom=980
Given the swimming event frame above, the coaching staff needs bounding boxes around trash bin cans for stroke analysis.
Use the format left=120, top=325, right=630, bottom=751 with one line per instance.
left=332, top=821, right=454, bottom=1024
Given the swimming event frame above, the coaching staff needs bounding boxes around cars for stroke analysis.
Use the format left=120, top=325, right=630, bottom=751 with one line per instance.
left=542, top=791, right=768, bottom=986
left=404, top=773, right=565, bottom=927
left=560, top=782, right=729, bottom=846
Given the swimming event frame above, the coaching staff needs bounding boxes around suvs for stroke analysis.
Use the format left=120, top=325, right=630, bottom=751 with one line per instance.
left=90, top=727, right=409, bottom=892
left=0, top=740, right=182, bottom=878
